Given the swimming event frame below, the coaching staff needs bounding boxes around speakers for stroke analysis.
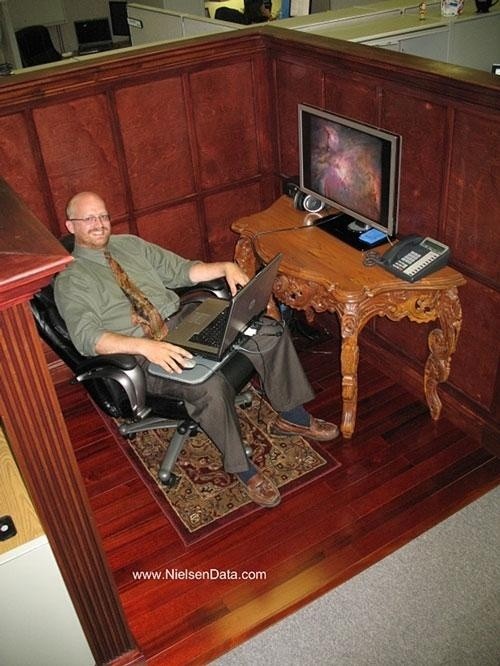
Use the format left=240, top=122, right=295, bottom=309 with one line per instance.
left=285, top=175, right=299, bottom=197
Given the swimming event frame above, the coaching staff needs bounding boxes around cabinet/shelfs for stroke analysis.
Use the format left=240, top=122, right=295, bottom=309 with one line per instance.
left=449, top=14, right=500, bottom=76
left=361, top=26, right=449, bottom=65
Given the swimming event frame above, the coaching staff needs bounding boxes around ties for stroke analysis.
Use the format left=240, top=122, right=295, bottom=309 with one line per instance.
left=105, top=252, right=168, bottom=341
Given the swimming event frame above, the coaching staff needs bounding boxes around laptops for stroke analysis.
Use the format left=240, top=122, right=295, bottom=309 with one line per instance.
left=160, top=250, right=285, bottom=363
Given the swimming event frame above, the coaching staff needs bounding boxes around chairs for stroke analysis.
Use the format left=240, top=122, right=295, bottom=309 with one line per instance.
left=24, top=233, right=255, bottom=487
left=214, top=6, right=250, bottom=25
left=15, top=25, right=62, bottom=68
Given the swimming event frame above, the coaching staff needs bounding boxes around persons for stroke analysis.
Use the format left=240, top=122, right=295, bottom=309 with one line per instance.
left=50, top=189, right=340, bottom=510
left=244, top=0, right=276, bottom=25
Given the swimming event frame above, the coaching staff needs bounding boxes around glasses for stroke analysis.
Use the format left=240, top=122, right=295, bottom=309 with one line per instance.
left=68, top=215, right=111, bottom=225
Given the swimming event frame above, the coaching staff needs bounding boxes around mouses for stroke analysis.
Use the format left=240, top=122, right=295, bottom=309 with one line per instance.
left=176, top=355, right=196, bottom=370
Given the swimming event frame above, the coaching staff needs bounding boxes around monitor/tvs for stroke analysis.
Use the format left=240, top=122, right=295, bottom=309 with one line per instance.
left=296, top=100, right=403, bottom=252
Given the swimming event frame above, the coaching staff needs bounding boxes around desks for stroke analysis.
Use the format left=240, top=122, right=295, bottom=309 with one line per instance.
left=231, top=196, right=468, bottom=440
left=0, top=427, right=97, bottom=666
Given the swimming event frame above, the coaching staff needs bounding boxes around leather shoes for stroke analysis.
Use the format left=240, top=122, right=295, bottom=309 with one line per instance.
left=234, top=457, right=281, bottom=508
left=272, top=414, right=340, bottom=441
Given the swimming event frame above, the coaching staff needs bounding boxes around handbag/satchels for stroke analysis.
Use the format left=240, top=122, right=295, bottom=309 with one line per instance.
left=276, top=299, right=330, bottom=351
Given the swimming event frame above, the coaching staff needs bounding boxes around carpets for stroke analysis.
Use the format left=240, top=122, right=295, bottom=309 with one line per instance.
left=80, top=381, right=343, bottom=549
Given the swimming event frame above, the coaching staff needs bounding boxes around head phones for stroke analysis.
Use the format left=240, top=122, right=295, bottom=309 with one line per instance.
left=293, top=191, right=325, bottom=213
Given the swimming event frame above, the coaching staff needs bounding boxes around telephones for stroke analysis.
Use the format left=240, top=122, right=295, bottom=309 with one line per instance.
left=381, top=232, right=451, bottom=283
